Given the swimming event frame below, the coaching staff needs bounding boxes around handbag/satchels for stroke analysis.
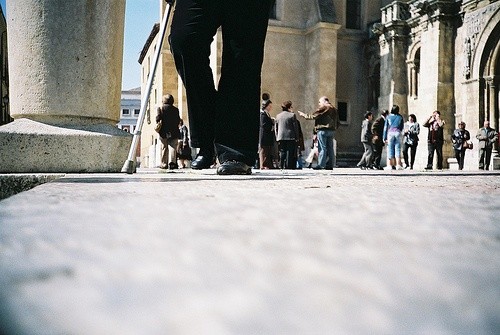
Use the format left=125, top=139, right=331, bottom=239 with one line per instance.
left=463, top=140, right=473, bottom=149
left=155, top=120, right=162, bottom=133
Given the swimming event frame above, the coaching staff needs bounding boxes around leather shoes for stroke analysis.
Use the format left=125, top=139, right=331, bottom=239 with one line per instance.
left=217, top=159, right=252, bottom=175
left=190, top=155, right=214, bottom=169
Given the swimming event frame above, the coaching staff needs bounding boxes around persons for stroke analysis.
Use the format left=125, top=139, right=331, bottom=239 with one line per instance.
left=357, top=110, right=375, bottom=169
left=451, top=121, right=470, bottom=170
left=251, top=92, right=340, bottom=170
left=423, top=110, right=446, bottom=170
left=476, top=121, right=498, bottom=170
left=368, top=109, right=391, bottom=170
left=166, top=0, right=276, bottom=176
left=383, top=104, right=404, bottom=170
left=400, top=113, right=420, bottom=170
left=175, top=119, right=220, bottom=172
left=154, top=93, right=182, bottom=170
